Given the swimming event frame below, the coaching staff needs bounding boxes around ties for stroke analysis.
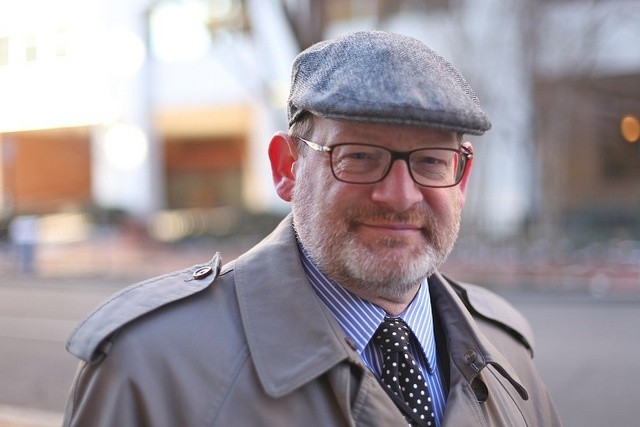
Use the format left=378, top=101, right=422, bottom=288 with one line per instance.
left=372, top=317, right=439, bottom=427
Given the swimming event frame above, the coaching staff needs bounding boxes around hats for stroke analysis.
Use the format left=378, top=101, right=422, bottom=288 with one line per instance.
left=286, top=30, right=494, bottom=139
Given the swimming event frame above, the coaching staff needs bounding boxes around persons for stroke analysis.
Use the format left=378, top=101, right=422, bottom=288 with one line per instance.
left=61, top=30, right=562, bottom=427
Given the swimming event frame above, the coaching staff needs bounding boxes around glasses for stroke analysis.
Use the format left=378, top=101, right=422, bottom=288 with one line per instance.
left=289, top=134, right=474, bottom=189
